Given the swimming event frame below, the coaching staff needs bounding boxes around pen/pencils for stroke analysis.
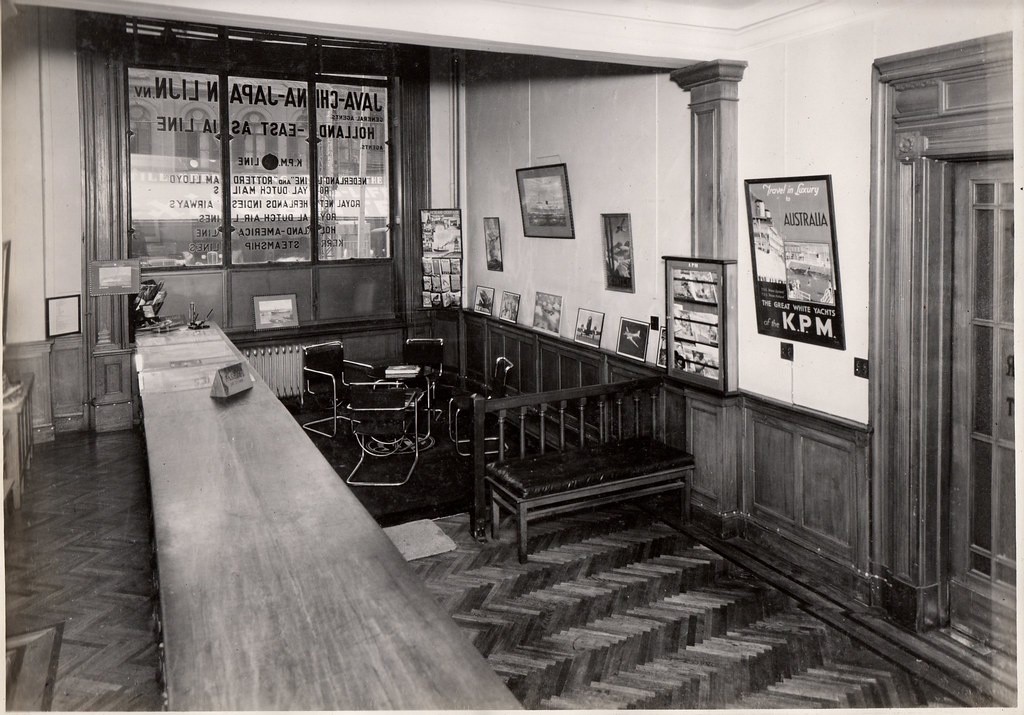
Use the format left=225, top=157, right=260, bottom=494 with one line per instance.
left=193, top=313, right=199, bottom=324
left=199, top=309, right=213, bottom=326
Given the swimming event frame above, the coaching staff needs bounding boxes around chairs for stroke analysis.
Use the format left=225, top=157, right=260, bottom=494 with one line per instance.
left=404, top=338, right=444, bottom=422
left=303, top=340, right=374, bottom=439
left=346, top=382, right=419, bottom=486
left=449, top=357, right=515, bottom=455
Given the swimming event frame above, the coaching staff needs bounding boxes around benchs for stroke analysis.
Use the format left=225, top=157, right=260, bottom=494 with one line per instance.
left=486, top=435, right=697, bottom=563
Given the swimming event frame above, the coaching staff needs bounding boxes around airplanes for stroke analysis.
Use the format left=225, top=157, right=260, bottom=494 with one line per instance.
left=623, top=326, right=641, bottom=348
left=476, top=290, right=492, bottom=313
left=577, top=316, right=600, bottom=339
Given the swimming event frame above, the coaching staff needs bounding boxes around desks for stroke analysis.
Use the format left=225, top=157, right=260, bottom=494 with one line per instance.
left=2, top=371, right=36, bottom=512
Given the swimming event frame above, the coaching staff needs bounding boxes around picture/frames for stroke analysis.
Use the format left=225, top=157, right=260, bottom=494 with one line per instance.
left=473, top=285, right=495, bottom=316
left=573, top=307, right=605, bottom=349
left=251, top=293, right=301, bottom=332
left=600, top=213, right=636, bottom=295
left=616, top=316, right=650, bottom=363
left=499, top=290, right=521, bottom=324
left=516, top=162, right=576, bottom=240
left=483, top=216, right=504, bottom=272
left=655, top=326, right=667, bottom=369
left=532, top=291, right=564, bottom=338
left=743, top=174, right=847, bottom=350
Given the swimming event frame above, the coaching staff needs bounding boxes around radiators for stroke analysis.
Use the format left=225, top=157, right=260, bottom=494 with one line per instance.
left=243, top=344, right=305, bottom=397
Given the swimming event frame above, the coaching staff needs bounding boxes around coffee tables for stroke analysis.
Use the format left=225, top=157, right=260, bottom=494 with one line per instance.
left=367, top=365, right=440, bottom=448
